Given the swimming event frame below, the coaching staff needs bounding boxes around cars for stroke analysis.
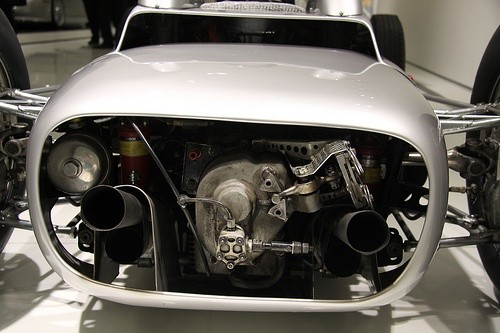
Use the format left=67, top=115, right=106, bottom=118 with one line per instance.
left=10, top=0, right=88, bottom=29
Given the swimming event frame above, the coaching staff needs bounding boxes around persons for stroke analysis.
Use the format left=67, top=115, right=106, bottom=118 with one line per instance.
left=82, top=0, right=137, bottom=48
